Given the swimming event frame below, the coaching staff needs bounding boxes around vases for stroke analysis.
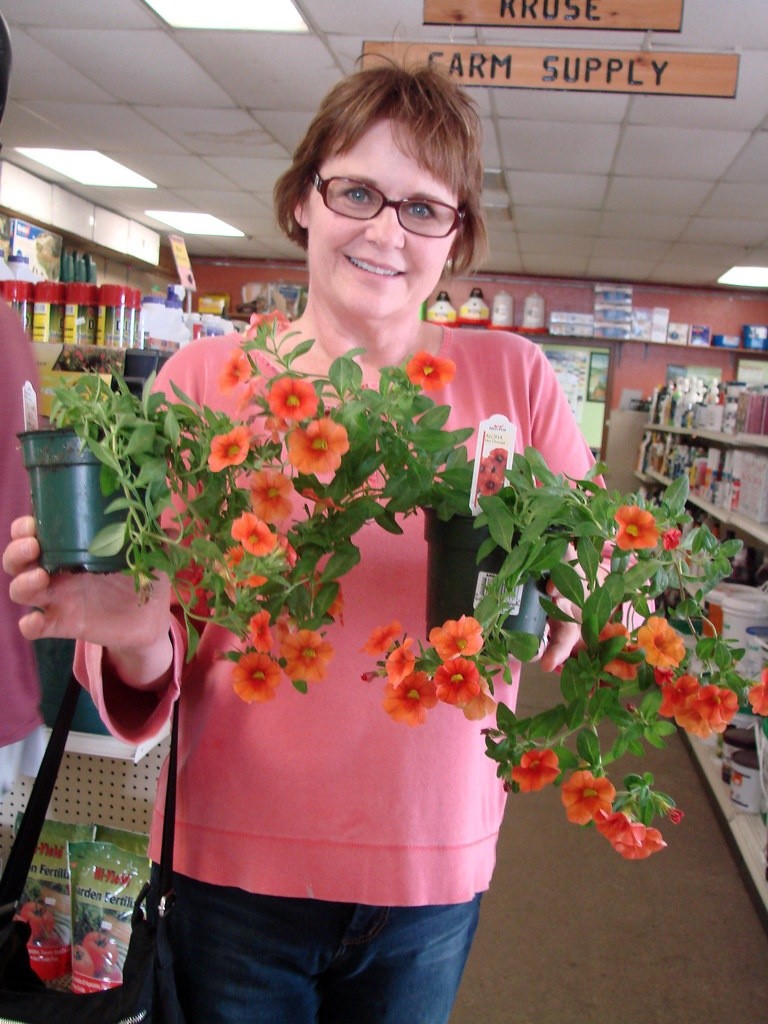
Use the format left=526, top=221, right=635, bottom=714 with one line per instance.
left=17, top=426, right=154, bottom=574
left=419, top=506, right=553, bottom=658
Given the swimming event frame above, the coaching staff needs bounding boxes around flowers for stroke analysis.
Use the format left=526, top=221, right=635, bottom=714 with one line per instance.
left=49, top=308, right=472, bottom=704
left=357, top=445, right=768, bottom=862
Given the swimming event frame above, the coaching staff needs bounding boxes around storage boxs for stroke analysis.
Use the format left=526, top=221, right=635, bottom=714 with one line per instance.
left=734, top=391, right=768, bottom=436
left=549, top=284, right=768, bottom=351
left=719, top=448, right=768, bottom=526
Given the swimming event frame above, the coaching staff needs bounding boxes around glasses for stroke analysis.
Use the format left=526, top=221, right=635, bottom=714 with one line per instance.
left=308, top=166, right=468, bottom=239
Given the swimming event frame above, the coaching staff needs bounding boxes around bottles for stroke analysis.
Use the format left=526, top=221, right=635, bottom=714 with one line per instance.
left=645, top=375, right=722, bottom=487
left=522, top=291, right=544, bottom=329
left=461, top=288, right=489, bottom=321
left=492, top=289, right=513, bottom=327
left=426, top=290, right=456, bottom=323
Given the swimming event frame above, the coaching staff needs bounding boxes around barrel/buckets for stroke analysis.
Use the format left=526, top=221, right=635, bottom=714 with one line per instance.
left=716, top=712, right=760, bottom=814
left=721, top=593, right=768, bottom=672
left=704, top=584, right=752, bottom=639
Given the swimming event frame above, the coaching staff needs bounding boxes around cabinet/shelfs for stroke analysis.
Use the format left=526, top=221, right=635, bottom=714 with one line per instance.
left=0, top=345, right=172, bottom=993
left=634, top=425, right=768, bottom=936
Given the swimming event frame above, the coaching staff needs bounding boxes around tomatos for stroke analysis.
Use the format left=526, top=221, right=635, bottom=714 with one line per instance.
left=12, top=901, right=54, bottom=944
left=71, top=930, right=121, bottom=976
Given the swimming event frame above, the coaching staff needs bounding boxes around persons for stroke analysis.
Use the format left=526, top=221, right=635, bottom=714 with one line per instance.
left=0, top=12, right=53, bottom=899
left=3, top=51, right=656, bottom=1024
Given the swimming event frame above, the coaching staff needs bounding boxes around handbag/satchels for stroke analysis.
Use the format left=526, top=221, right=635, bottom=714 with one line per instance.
left=1, top=673, right=178, bottom=1024
